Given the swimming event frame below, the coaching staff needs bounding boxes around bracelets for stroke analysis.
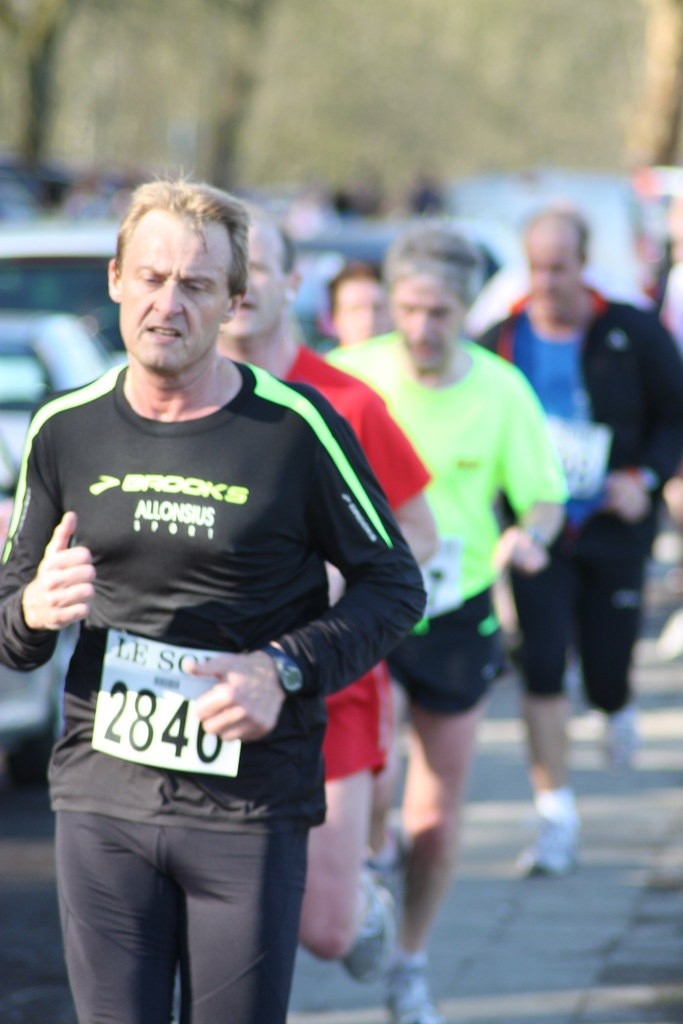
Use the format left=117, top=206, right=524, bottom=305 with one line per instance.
left=629, top=465, right=657, bottom=492
left=528, top=528, right=547, bottom=543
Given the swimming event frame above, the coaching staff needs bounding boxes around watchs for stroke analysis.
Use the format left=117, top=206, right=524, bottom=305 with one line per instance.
left=262, top=645, right=303, bottom=701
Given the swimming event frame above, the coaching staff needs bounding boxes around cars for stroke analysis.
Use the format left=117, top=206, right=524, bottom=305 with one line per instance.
left=0, top=160, right=682, bottom=753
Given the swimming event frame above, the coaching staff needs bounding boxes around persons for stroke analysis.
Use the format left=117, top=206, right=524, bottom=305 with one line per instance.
left=216, top=216, right=439, bottom=982
left=321, top=234, right=570, bottom=1024
left=327, top=262, right=397, bottom=869
left=0, top=181, right=429, bottom=1024
left=472, top=211, right=683, bottom=876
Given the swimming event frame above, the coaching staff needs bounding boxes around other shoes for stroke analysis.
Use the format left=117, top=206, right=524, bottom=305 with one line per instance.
left=603, top=715, right=632, bottom=770
left=386, top=964, right=435, bottom=1024
left=345, top=871, right=394, bottom=984
left=518, top=820, right=581, bottom=876
